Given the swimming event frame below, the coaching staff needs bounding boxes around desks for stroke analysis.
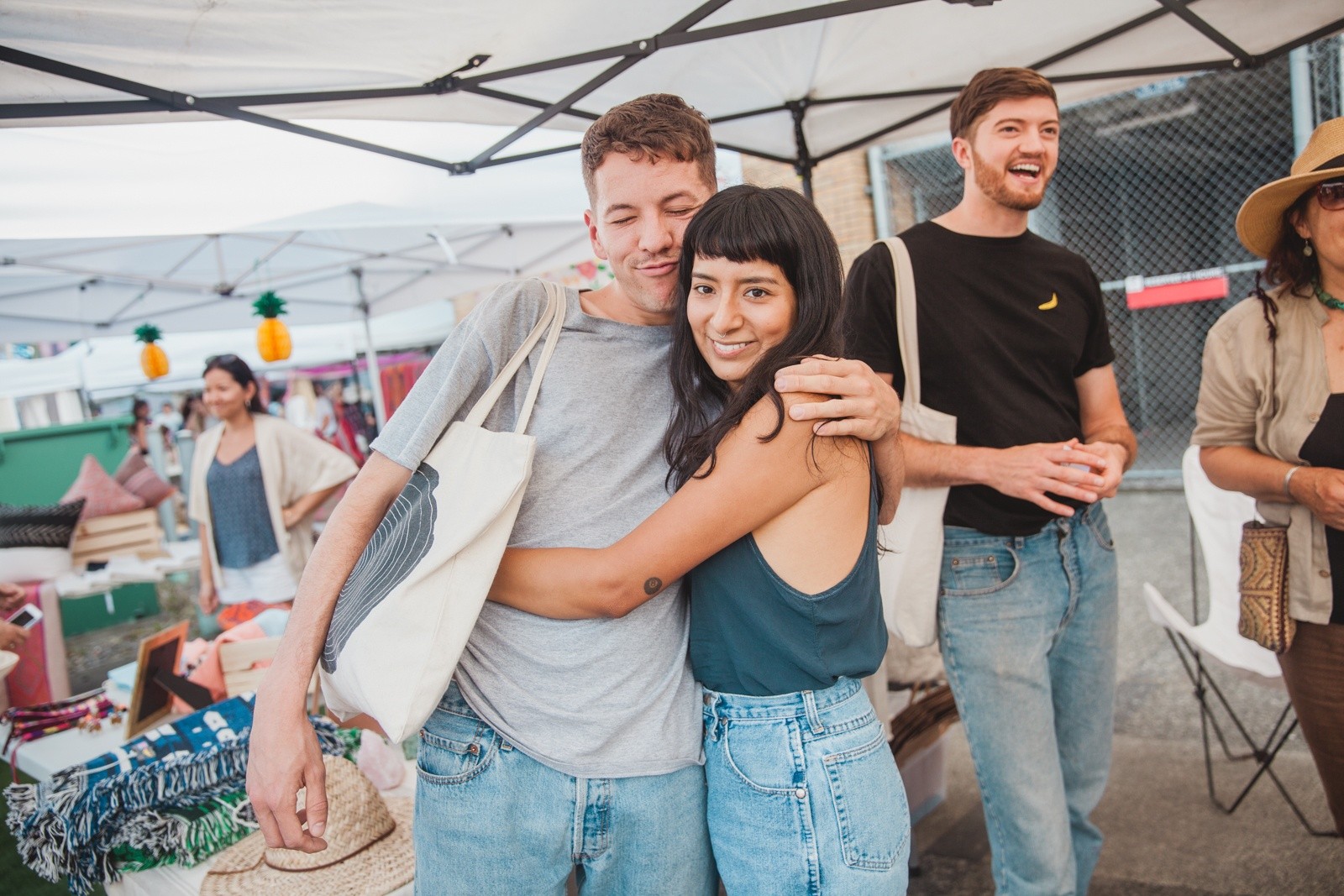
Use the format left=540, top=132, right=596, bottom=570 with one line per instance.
left=0, top=642, right=960, bottom=896
left=53, top=538, right=199, bottom=600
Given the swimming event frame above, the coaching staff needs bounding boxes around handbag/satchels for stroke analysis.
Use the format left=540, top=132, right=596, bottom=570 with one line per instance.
left=867, top=235, right=959, bottom=649
left=315, top=275, right=568, bottom=744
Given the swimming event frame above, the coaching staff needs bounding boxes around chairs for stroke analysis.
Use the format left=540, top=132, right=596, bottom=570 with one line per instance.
left=1142, top=444, right=1337, bottom=838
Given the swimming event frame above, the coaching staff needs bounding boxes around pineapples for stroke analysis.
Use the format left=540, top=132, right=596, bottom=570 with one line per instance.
left=251, top=289, right=291, bottom=361
left=134, top=324, right=169, bottom=379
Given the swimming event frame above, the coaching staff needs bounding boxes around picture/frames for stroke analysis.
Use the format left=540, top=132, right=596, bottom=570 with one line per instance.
left=125, top=619, right=189, bottom=739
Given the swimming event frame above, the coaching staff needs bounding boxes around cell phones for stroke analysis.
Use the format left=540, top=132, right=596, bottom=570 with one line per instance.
left=7, top=602, right=43, bottom=630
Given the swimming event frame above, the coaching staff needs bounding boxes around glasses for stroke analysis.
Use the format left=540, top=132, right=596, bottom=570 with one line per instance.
left=1314, top=179, right=1344, bottom=211
left=205, top=354, right=249, bottom=373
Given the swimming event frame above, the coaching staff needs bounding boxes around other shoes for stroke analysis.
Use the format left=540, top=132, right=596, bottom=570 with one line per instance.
left=1237, top=520, right=1298, bottom=656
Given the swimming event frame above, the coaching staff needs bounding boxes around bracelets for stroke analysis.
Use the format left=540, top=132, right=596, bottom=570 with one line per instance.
left=1282, top=465, right=1303, bottom=503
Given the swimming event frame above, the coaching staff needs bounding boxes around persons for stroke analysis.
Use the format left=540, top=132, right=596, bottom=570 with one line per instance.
left=0, top=581, right=28, bottom=648
left=245, top=94, right=906, bottom=896
left=485, top=183, right=914, bottom=896
left=844, top=68, right=1139, bottom=896
left=123, top=353, right=361, bottom=632
left=1192, top=114, right=1344, bottom=843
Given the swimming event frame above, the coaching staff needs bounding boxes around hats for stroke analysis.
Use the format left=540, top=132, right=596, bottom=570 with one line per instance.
left=201, top=753, right=417, bottom=896
left=1235, top=115, right=1344, bottom=261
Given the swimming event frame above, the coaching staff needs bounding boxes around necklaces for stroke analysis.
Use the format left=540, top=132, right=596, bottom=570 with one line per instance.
left=1310, top=275, right=1344, bottom=310
